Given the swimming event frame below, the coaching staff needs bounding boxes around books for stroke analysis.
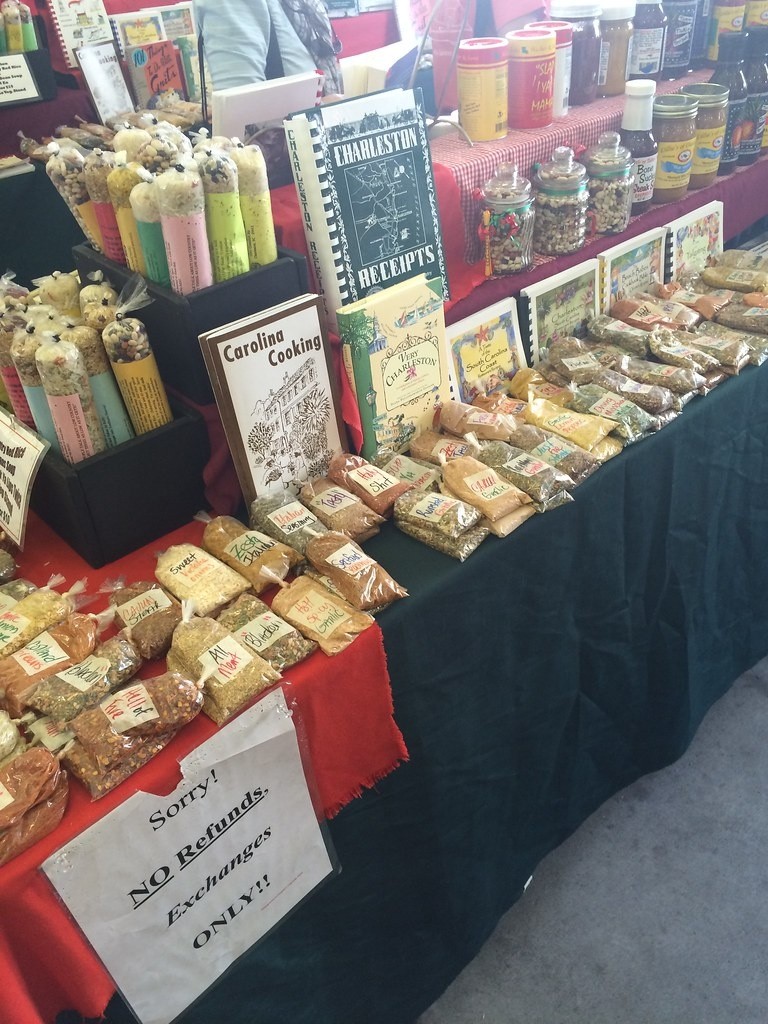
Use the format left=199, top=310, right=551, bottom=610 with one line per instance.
left=274, top=90, right=464, bottom=330
left=198, top=291, right=347, bottom=519
left=600, top=227, right=667, bottom=317
left=521, top=258, right=600, bottom=366
left=336, top=273, right=464, bottom=460
left=664, top=199, right=723, bottom=286
left=436, top=296, right=526, bottom=394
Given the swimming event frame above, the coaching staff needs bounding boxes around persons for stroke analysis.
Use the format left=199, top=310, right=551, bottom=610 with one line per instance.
left=406, top=0, right=554, bottom=120
left=189, top=0, right=360, bottom=184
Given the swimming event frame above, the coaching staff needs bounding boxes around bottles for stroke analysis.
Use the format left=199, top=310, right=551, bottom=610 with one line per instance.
left=706, top=31, right=748, bottom=176
left=735, top=24, right=768, bottom=166
left=532, top=146, right=591, bottom=256
left=477, top=161, right=536, bottom=276
left=458, top=0, right=768, bottom=141
left=579, top=130, right=636, bottom=235
left=619, top=78, right=658, bottom=216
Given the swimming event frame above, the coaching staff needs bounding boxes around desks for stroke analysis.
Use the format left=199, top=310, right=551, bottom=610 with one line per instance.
left=0, top=223, right=768, bottom=1024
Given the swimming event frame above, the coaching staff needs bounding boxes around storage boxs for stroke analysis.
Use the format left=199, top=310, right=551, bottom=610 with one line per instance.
left=0, top=15, right=57, bottom=108
left=29, top=394, right=212, bottom=568
left=70, top=242, right=310, bottom=406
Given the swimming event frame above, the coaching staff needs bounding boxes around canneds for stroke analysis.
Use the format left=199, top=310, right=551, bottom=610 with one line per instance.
left=652, top=82, right=731, bottom=205
left=549, top=0, right=668, bottom=106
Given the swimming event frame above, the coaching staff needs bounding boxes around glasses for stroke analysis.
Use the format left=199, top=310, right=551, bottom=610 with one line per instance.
left=312, top=31, right=343, bottom=58
